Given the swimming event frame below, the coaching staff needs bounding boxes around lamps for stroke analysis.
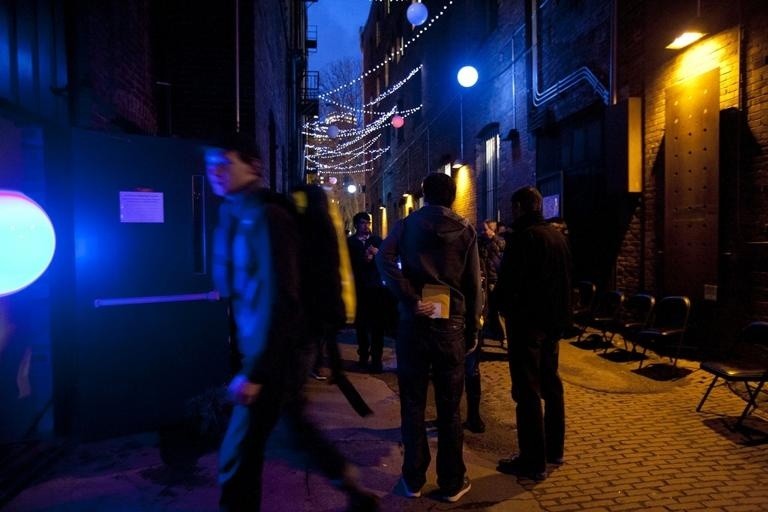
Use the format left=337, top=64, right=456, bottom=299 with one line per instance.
left=391, top=108, right=404, bottom=128
left=403, top=191, right=411, bottom=197
left=327, top=122, right=339, bottom=139
left=452, top=159, right=464, bottom=169
left=406, top=0, right=428, bottom=26
left=656, top=0, right=710, bottom=50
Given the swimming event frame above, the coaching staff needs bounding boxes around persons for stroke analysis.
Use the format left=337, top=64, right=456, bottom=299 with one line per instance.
left=485, top=185, right=572, bottom=481
left=203, top=134, right=381, bottom=511
left=374, top=171, right=473, bottom=502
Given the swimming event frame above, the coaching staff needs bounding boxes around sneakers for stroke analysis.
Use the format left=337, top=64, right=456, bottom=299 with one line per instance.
left=400, top=471, right=423, bottom=498
left=497, top=456, right=565, bottom=481
left=438, top=476, right=473, bottom=502
left=310, top=366, right=328, bottom=380
left=358, top=352, right=384, bottom=374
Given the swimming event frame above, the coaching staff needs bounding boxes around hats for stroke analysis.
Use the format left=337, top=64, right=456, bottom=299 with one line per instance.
left=423, top=173, right=457, bottom=203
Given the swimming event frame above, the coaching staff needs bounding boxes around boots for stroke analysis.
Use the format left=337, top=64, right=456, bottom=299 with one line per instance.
left=466, top=394, right=486, bottom=433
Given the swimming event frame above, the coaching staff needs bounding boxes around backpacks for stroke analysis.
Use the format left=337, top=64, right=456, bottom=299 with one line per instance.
left=244, top=185, right=357, bottom=345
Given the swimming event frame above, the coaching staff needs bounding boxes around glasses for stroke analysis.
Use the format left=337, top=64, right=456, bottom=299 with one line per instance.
left=356, top=221, right=372, bottom=225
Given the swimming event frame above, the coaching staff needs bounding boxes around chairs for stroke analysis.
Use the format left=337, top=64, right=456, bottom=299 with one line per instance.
left=573, top=281, right=768, bottom=433
